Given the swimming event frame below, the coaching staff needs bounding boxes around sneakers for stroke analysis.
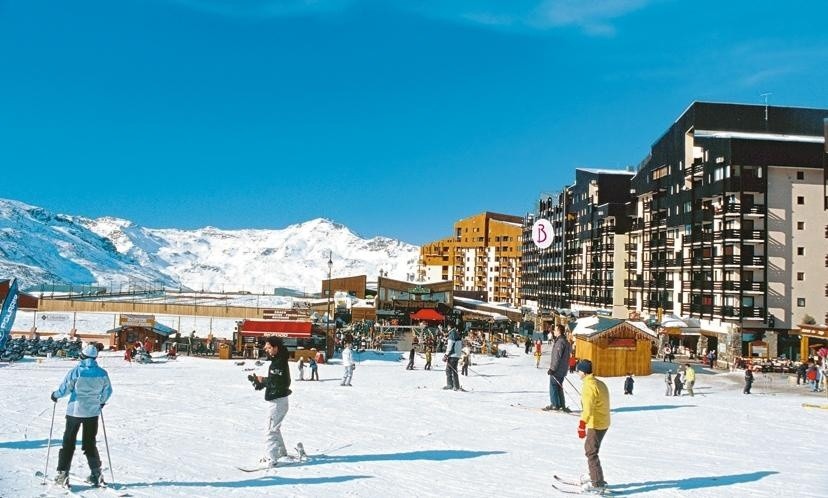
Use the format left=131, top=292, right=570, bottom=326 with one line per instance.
left=54, top=471, right=69, bottom=485
left=581, top=482, right=604, bottom=493
left=86, top=467, right=103, bottom=484
left=546, top=405, right=559, bottom=410
left=443, top=385, right=453, bottom=389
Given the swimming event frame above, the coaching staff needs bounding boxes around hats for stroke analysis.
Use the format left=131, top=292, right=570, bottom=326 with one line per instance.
left=577, top=359, right=592, bottom=373
left=79, top=346, right=98, bottom=361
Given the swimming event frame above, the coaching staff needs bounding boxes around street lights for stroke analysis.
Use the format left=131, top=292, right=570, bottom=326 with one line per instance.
left=323, top=251, right=334, bottom=362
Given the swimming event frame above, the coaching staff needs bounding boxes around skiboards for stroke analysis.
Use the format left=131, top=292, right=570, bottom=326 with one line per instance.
left=37, top=471, right=132, bottom=497
left=236, top=454, right=325, bottom=472
left=511, top=404, right=582, bottom=417
left=552, top=475, right=627, bottom=498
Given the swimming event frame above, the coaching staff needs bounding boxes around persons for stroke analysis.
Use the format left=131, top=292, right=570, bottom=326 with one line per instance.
left=623, top=375, right=635, bottom=394
left=144, top=336, right=152, bottom=354
left=525, top=338, right=530, bottom=354
left=796, top=356, right=822, bottom=393
left=52, top=344, right=113, bottom=486
left=546, top=323, right=572, bottom=410
left=340, top=341, right=354, bottom=386
left=743, top=365, right=754, bottom=393
left=189, top=330, right=196, bottom=345
left=708, top=350, right=715, bottom=368
left=248, top=336, right=290, bottom=465
left=460, top=351, right=469, bottom=376
left=195, top=339, right=204, bottom=356
left=534, top=339, right=542, bottom=368
left=662, top=344, right=672, bottom=363
left=406, top=347, right=416, bottom=370
left=425, top=347, right=432, bottom=370
left=296, top=356, right=307, bottom=380
left=441, top=322, right=462, bottom=391
left=576, top=359, right=611, bottom=492
left=131, top=340, right=141, bottom=358
left=242, top=343, right=250, bottom=359
left=167, top=341, right=177, bottom=358
left=205, top=340, right=216, bottom=356
left=306, top=356, right=319, bottom=381
left=664, top=364, right=696, bottom=397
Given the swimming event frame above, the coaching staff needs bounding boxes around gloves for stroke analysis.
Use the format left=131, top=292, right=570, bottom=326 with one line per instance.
left=442, top=354, right=449, bottom=362
left=248, top=373, right=261, bottom=390
left=578, top=420, right=586, bottom=438
left=51, top=392, right=57, bottom=403
left=547, top=369, right=555, bottom=375
